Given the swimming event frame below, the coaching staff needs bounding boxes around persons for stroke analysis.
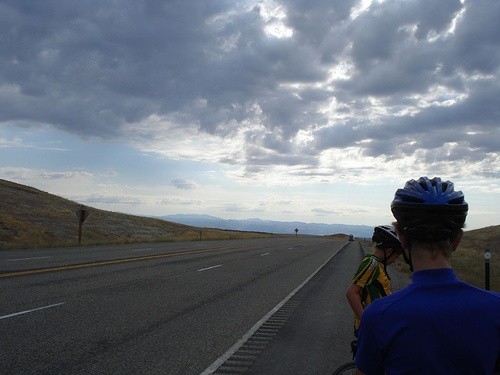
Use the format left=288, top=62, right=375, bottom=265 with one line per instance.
left=345, top=224, right=403, bottom=339
left=355, top=176, right=500, bottom=375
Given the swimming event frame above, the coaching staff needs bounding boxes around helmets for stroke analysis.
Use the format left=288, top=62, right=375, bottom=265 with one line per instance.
left=373, top=226, right=401, bottom=243
left=391, top=177, right=468, bottom=229
